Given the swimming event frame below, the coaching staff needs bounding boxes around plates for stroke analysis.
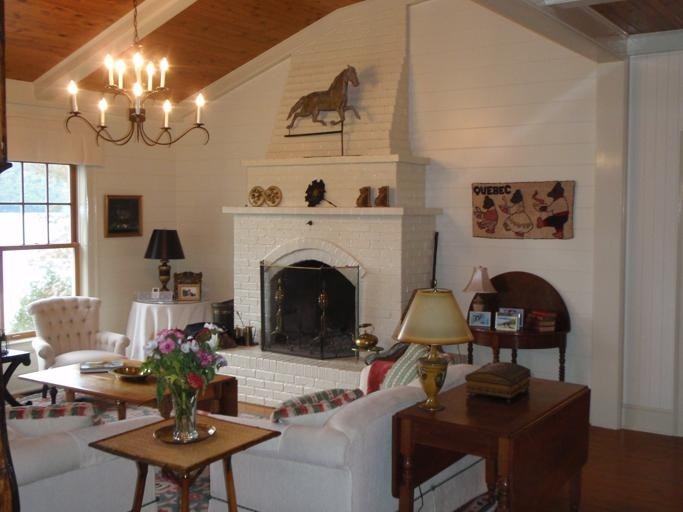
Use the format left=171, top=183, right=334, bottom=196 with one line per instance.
left=248, top=186, right=282, bottom=207
left=153, top=423, right=217, bottom=446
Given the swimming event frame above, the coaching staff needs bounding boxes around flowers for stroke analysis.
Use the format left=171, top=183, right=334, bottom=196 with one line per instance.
left=138, top=320, right=227, bottom=420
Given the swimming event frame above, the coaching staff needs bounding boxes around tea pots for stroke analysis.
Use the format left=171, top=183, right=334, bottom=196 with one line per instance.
left=349, top=323, right=379, bottom=347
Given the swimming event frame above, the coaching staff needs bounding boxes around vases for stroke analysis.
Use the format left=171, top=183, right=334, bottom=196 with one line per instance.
left=169, top=388, right=199, bottom=441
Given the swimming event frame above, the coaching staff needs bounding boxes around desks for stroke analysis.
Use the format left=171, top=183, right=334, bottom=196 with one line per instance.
left=389, top=377, right=593, bottom=511
left=1, top=348, right=32, bottom=406
left=88, top=414, right=280, bottom=511
left=17, top=356, right=241, bottom=424
left=464, top=272, right=571, bottom=383
left=124, top=295, right=218, bottom=361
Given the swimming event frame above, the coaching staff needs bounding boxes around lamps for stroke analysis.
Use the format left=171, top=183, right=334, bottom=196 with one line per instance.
left=63, top=0, right=212, bottom=150
left=143, top=228, right=187, bottom=289
left=397, top=277, right=475, bottom=413
left=461, top=265, right=497, bottom=312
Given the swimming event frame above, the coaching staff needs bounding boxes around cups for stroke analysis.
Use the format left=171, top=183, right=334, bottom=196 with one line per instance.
left=242, top=326, right=256, bottom=346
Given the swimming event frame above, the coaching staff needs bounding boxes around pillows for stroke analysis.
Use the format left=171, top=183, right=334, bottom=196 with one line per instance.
left=378, top=341, right=432, bottom=390
left=8, top=401, right=98, bottom=420
left=270, top=388, right=364, bottom=423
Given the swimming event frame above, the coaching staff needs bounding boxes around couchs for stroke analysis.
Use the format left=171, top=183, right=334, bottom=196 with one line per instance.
left=0, top=401, right=165, bottom=511
left=204, top=360, right=493, bottom=512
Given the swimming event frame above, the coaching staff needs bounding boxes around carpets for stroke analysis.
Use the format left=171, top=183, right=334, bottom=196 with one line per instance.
left=14, top=398, right=500, bottom=512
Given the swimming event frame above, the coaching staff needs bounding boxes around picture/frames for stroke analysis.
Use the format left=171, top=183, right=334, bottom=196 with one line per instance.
left=102, top=192, right=144, bottom=240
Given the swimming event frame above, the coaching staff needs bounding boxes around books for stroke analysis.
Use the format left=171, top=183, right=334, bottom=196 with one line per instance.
left=79, top=359, right=124, bottom=375
left=525, top=309, right=557, bottom=334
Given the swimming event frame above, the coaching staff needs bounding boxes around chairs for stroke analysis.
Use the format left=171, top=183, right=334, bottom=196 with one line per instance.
left=27, top=295, right=131, bottom=407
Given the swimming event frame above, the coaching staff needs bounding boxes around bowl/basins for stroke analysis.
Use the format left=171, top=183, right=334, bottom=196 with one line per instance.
left=107, top=366, right=152, bottom=381
